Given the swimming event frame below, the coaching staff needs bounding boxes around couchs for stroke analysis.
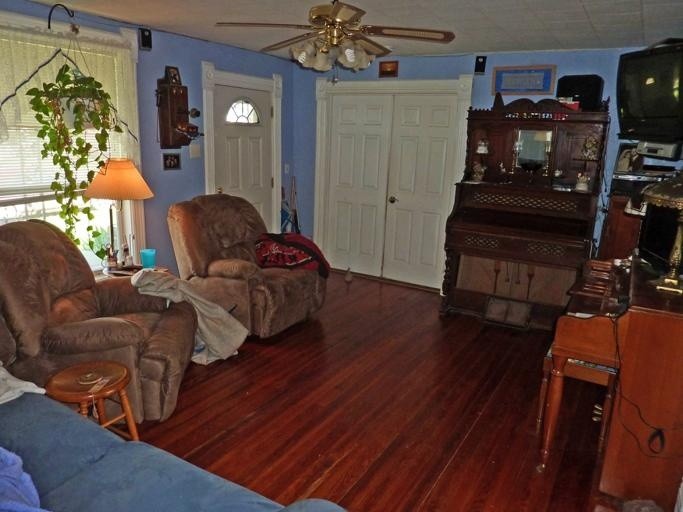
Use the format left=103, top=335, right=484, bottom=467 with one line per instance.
left=0, top=393, right=350, bottom=512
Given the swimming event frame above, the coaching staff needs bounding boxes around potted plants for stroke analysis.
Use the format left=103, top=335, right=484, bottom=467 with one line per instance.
left=26, top=64, right=138, bottom=260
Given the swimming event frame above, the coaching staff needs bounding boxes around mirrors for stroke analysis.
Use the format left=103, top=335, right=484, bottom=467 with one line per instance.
left=511, top=124, right=558, bottom=176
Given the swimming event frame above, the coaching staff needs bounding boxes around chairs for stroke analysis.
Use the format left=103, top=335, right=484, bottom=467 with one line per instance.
left=166, top=194, right=328, bottom=339
left=0, top=219, right=198, bottom=424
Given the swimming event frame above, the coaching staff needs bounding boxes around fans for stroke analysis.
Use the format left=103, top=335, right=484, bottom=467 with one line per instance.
left=215, top=1, right=455, bottom=57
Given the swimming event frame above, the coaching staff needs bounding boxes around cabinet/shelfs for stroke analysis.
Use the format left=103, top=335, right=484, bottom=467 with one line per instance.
left=535, top=257, right=683, bottom=512
left=438, top=92, right=610, bottom=333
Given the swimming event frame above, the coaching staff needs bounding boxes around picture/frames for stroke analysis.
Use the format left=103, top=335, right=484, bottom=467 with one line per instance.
left=379, top=61, right=399, bottom=77
left=492, top=65, right=556, bottom=95
left=614, top=143, right=645, bottom=176
left=163, top=153, right=182, bottom=170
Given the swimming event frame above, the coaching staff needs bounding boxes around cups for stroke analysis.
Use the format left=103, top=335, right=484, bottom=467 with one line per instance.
left=574, top=170, right=591, bottom=193
left=139, top=248, right=156, bottom=269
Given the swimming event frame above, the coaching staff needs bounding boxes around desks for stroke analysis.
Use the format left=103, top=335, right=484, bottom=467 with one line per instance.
left=103, top=264, right=168, bottom=278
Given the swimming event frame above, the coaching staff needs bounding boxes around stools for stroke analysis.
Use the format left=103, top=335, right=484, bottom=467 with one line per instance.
left=44, top=360, right=140, bottom=441
left=536, top=346, right=553, bottom=435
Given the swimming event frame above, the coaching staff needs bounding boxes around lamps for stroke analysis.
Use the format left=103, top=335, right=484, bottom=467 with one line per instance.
left=642, top=167, right=683, bottom=294
left=290, top=28, right=376, bottom=82
left=84, top=158, right=154, bottom=272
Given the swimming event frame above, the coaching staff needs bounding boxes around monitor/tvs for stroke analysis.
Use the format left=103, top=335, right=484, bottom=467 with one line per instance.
left=616, top=43, right=683, bottom=136
left=639, top=201, right=683, bottom=276
